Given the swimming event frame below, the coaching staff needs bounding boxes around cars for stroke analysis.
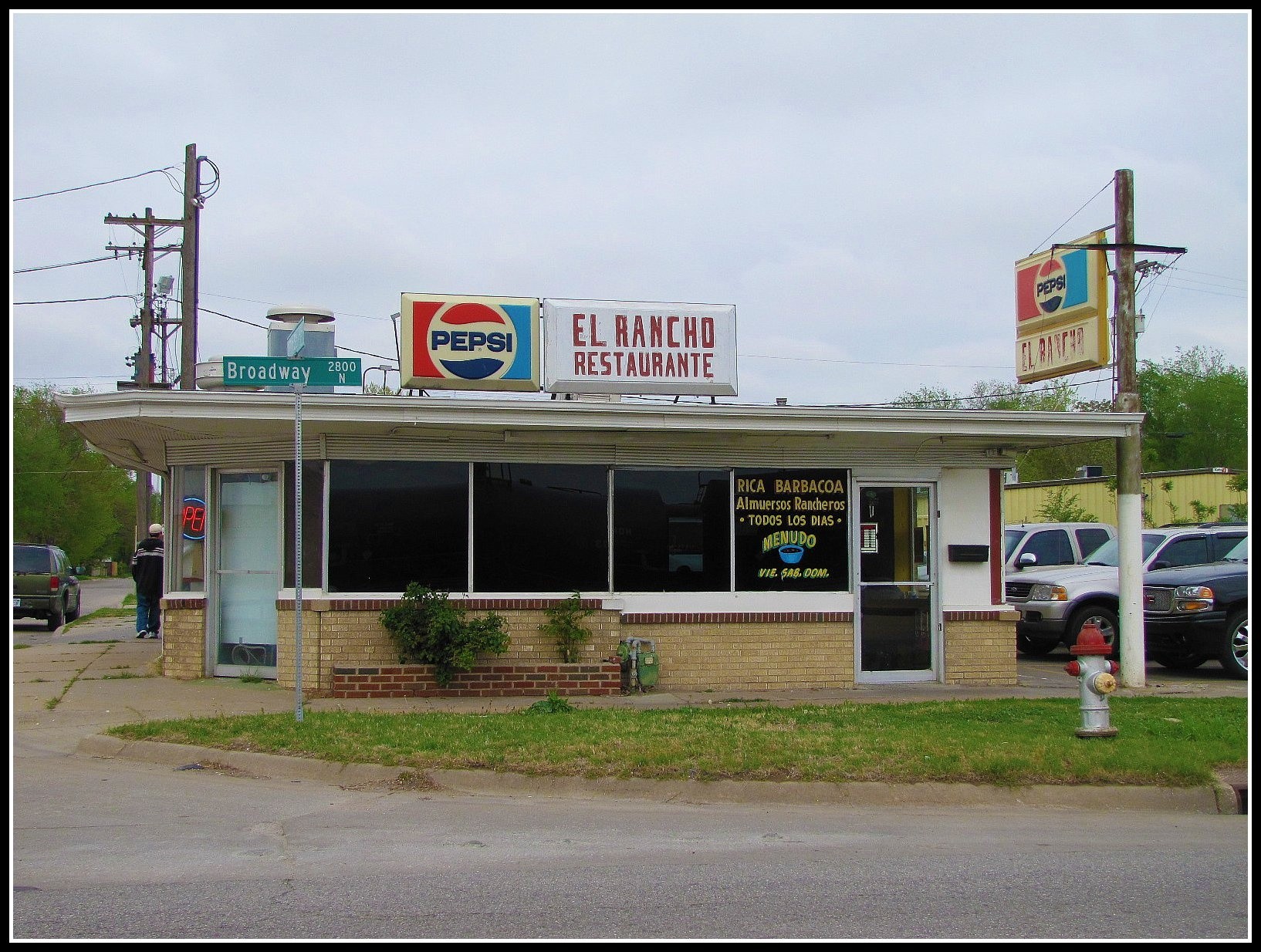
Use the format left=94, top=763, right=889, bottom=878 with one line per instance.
left=1117, top=535, right=1249, bottom=681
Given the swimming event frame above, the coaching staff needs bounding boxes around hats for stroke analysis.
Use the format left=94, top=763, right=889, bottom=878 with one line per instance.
left=149, top=523, right=165, bottom=534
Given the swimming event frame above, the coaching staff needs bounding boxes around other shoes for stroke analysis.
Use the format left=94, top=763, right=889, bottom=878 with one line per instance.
left=136, top=630, right=147, bottom=638
left=149, top=632, right=158, bottom=639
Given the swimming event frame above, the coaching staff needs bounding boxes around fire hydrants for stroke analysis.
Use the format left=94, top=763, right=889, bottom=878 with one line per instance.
left=1063, top=622, right=1121, bottom=740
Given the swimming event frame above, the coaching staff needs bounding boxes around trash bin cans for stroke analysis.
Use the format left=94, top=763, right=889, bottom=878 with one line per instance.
left=74, top=567, right=84, bottom=575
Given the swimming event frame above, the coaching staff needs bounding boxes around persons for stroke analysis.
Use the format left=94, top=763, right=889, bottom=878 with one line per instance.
left=132, top=523, right=164, bottom=638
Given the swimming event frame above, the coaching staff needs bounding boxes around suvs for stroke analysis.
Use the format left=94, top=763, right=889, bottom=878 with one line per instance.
left=1004, top=521, right=1248, bottom=662
left=13, top=542, right=81, bottom=633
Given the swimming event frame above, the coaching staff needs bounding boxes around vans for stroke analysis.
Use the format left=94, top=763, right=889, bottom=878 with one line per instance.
left=1003, top=522, right=1118, bottom=576
